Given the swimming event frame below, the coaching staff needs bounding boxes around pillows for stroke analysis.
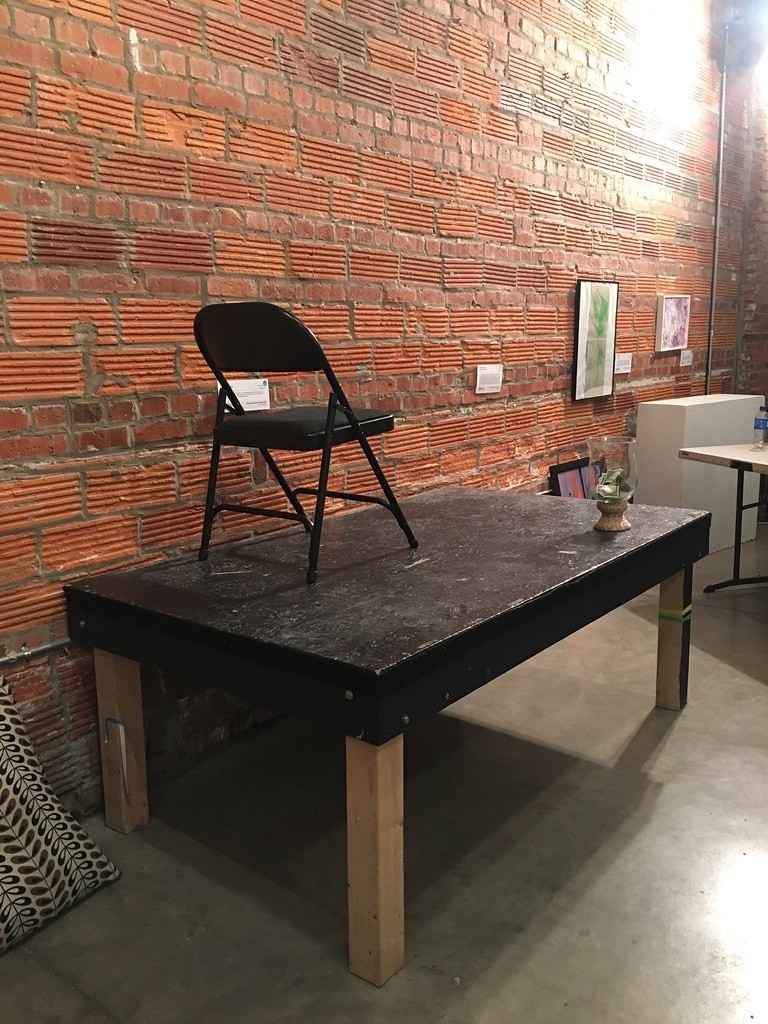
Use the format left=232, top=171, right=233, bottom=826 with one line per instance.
left=0, top=674, right=122, bottom=958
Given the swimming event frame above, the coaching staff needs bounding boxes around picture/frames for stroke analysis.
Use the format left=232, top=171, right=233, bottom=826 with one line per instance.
left=655, top=295, right=690, bottom=352
left=571, top=279, right=619, bottom=401
left=549, top=454, right=607, bottom=497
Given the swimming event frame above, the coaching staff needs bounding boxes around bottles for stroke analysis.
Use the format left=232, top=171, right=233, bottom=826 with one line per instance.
left=754, top=406, right=768, bottom=447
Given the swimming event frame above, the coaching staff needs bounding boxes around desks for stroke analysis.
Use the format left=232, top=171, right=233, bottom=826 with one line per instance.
left=678, top=443, right=768, bottom=592
left=61, top=485, right=712, bottom=986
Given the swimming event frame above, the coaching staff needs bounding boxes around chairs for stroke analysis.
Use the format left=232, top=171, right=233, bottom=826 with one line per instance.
left=193, top=302, right=419, bottom=584
